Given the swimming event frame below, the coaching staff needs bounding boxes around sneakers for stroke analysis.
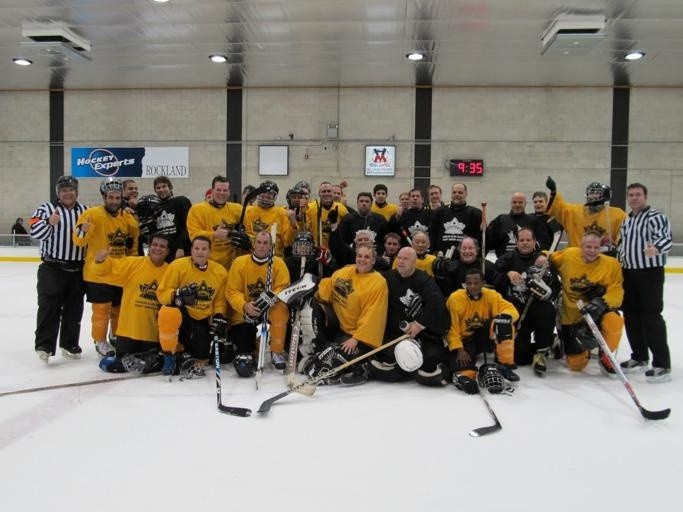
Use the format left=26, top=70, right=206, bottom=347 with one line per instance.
left=271, top=351, right=286, bottom=370
left=646, top=366, right=671, bottom=378
left=599, top=346, right=618, bottom=374
left=95, top=340, right=114, bottom=357
left=620, top=358, right=648, bottom=369
left=499, top=364, right=519, bottom=381
left=108, top=333, right=117, bottom=346
left=35, top=344, right=52, bottom=357
left=534, top=346, right=548, bottom=374
left=62, top=344, right=82, bottom=355
left=550, top=333, right=561, bottom=359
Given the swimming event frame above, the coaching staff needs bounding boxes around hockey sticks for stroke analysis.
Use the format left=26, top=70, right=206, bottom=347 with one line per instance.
left=213, top=334, right=252, bottom=417
left=254, top=224, right=277, bottom=390
left=514, top=214, right=563, bottom=339
left=576, top=298, right=671, bottom=420
left=285, top=256, right=317, bottom=397
left=257, top=333, right=412, bottom=413
left=229, top=185, right=268, bottom=267
left=468, top=390, right=503, bottom=438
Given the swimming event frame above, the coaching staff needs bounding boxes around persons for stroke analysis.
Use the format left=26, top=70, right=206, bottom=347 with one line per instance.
left=308, top=181, right=349, bottom=247
left=528, top=191, right=564, bottom=253
left=479, top=192, right=553, bottom=257
left=494, top=228, right=563, bottom=375
left=352, top=228, right=388, bottom=273
left=426, top=186, right=445, bottom=210
left=446, top=268, right=520, bottom=393
left=225, top=231, right=291, bottom=374
left=333, top=185, right=356, bottom=213
left=601, top=183, right=673, bottom=383
left=123, top=179, right=144, bottom=255
left=138, top=176, right=192, bottom=263
left=328, top=192, right=389, bottom=265
left=155, top=236, right=228, bottom=375
left=187, top=176, right=255, bottom=270
left=284, top=235, right=336, bottom=376
left=242, top=185, right=257, bottom=206
left=12, top=217, right=31, bottom=246
left=301, top=189, right=311, bottom=205
left=396, top=188, right=432, bottom=231
left=372, top=184, right=399, bottom=220
left=434, top=236, right=495, bottom=295
left=387, top=193, right=410, bottom=229
left=544, top=176, right=628, bottom=248
left=382, top=233, right=402, bottom=263
left=242, top=181, right=299, bottom=257
left=205, top=187, right=214, bottom=202
left=29, top=176, right=92, bottom=365
left=428, top=183, right=482, bottom=259
left=74, top=176, right=139, bottom=357
left=391, top=232, right=435, bottom=276
left=315, top=244, right=388, bottom=386
left=534, top=232, right=626, bottom=379
left=283, top=187, right=312, bottom=232
left=371, top=248, right=450, bottom=388
left=90, top=233, right=184, bottom=374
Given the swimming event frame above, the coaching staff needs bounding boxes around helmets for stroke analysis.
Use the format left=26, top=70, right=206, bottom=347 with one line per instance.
left=233, top=351, right=255, bottom=378
left=286, top=186, right=304, bottom=208
left=585, top=181, right=612, bottom=206
left=121, top=349, right=165, bottom=376
left=481, top=363, right=501, bottom=387
left=292, top=231, right=313, bottom=256
left=394, top=338, right=424, bottom=373
left=257, top=180, right=279, bottom=208
left=295, top=181, right=310, bottom=193
left=55, top=175, right=78, bottom=197
left=100, top=176, right=123, bottom=195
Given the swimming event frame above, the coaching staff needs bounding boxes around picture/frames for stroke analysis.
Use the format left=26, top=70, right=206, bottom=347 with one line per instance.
left=364, top=144, right=396, bottom=177
left=258, top=145, right=290, bottom=176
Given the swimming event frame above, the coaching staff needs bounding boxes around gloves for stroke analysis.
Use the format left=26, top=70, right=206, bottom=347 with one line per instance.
left=328, top=205, right=338, bottom=224
left=209, top=315, right=226, bottom=343
left=493, top=313, right=513, bottom=344
left=313, top=243, right=331, bottom=265
left=245, top=289, right=275, bottom=320
left=525, top=273, right=552, bottom=301
left=175, top=283, right=198, bottom=306
left=575, top=296, right=607, bottom=328
left=230, top=223, right=251, bottom=251
